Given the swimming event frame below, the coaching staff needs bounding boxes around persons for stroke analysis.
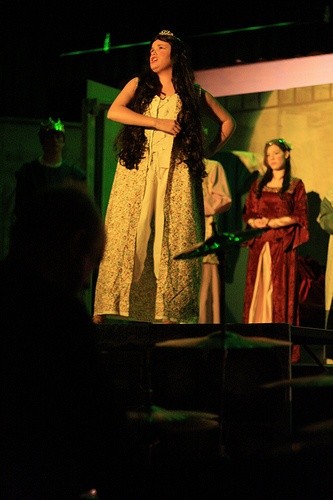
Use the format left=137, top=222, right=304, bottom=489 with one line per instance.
left=242, top=139, right=310, bottom=365
left=94, top=30, right=236, bottom=324
left=316, top=191, right=333, bottom=366
left=15, top=114, right=87, bottom=186
left=1, top=186, right=124, bottom=499
left=194, top=157, right=232, bottom=324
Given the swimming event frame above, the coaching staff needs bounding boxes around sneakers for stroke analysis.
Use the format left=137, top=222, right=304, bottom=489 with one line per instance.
left=92, top=316, right=108, bottom=324
left=325, top=359, right=333, bottom=364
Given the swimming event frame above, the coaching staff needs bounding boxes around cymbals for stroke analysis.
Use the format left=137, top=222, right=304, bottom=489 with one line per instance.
left=154, top=330, right=293, bottom=351
left=259, top=369, right=333, bottom=389
left=120, top=405, right=220, bottom=434
left=173, top=227, right=272, bottom=260
left=298, top=420, right=333, bottom=435
